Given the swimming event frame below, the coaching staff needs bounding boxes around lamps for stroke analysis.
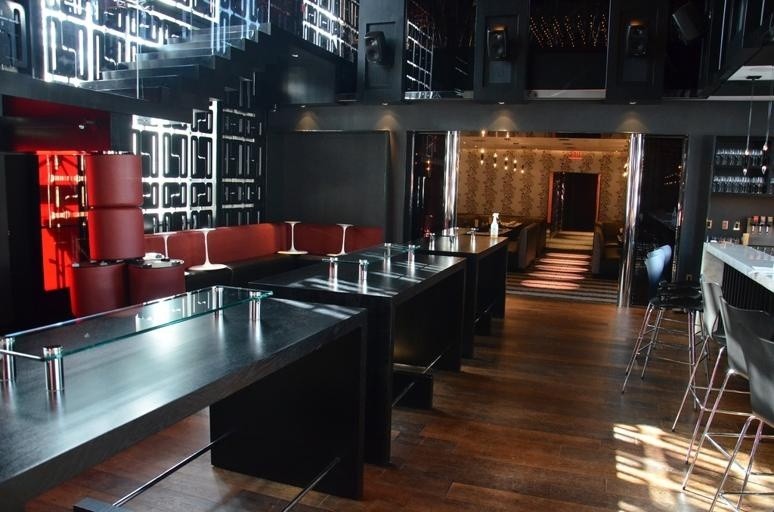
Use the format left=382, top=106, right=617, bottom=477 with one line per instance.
left=625, top=24, right=649, bottom=59
left=487, top=28, right=512, bottom=62
left=364, top=30, right=391, bottom=65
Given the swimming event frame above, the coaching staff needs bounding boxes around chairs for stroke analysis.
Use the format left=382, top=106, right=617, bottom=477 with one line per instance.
left=590, top=219, right=621, bottom=279
left=621, top=243, right=774, bottom=511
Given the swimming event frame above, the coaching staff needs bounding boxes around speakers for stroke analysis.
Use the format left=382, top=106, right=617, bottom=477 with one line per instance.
left=623, top=24, right=645, bottom=58
left=487, top=29, right=508, bottom=58
left=364, top=30, right=387, bottom=64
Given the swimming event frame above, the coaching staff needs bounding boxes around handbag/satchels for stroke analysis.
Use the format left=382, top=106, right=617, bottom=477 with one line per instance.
left=657, top=279, right=702, bottom=306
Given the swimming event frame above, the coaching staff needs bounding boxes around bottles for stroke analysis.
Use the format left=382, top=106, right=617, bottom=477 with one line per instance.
left=715, top=149, right=764, bottom=167
left=713, top=176, right=764, bottom=194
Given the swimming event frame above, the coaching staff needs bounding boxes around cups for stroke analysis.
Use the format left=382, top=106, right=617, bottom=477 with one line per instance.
left=743, top=232, right=749, bottom=246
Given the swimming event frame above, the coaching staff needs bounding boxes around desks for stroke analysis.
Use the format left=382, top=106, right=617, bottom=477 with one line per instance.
left=0, top=215, right=547, bottom=512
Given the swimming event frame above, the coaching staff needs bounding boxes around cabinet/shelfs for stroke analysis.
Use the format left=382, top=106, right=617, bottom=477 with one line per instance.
left=709, top=136, right=773, bottom=196
